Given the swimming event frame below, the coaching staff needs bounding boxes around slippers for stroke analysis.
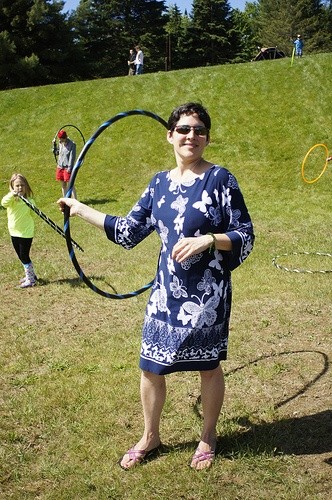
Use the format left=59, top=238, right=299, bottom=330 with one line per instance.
left=190, top=448, right=215, bottom=470
left=118, top=441, right=162, bottom=470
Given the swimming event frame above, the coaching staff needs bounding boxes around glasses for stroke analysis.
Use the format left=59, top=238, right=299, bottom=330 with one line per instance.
left=175, top=125, right=208, bottom=135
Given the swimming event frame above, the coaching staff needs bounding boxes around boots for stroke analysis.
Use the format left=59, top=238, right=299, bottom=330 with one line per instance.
left=20, top=261, right=35, bottom=288
left=20, top=275, right=37, bottom=283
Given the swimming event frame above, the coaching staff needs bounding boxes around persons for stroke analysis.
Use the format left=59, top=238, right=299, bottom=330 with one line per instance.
left=133, top=46, right=144, bottom=75
left=128, top=48, right=137, bottom=76
left=294, top=34, right=303, bottom=56
left=1, top=173, right=38, bottom=288
left=56, top=102, right=255, bottom=471
left=52, top=130, right=77, bottom=201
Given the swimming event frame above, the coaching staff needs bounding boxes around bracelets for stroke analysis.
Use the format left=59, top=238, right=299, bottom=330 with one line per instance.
left=206, top=232, right=216, bottom=253
